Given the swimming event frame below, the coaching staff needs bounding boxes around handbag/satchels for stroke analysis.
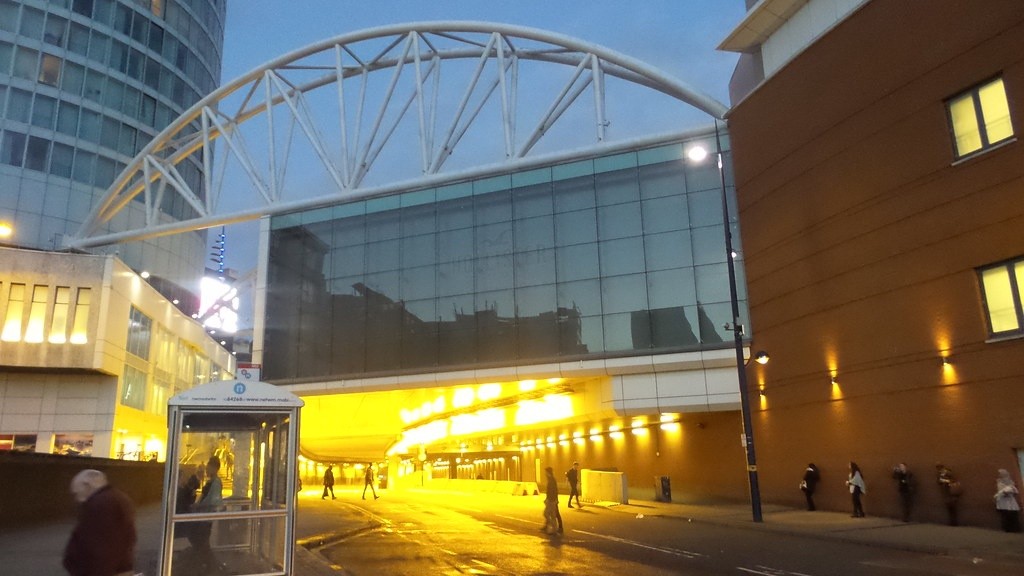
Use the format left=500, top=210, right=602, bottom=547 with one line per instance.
left=799, top=481, right=807, bottom=490
left=949, top=481, right=962, bottom=495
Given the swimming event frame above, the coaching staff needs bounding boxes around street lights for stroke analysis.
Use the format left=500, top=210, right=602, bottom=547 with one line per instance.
left=687, top=116, right=770, bottom=522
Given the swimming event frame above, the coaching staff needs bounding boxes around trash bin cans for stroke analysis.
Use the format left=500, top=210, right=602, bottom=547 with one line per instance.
left=215, top=495, right=252, bottom=545
left=653, top=474, right=671, bottom=502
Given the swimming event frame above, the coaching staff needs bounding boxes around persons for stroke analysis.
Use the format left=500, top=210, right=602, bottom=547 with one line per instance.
left=892, top=463, right=916, bottom=520
left=846, top=461, right=866, bottom=517
left=224, top=452, right=234, bottom=479
left=996, top=470, right=1021, bottom=532
left=362, top=462, right=380, bottom=500
left=62, top=470, right=138, bottom=576
left=173, top=457, right=222, bottom=537
left=322, top=465, right=337, bottom=499
left=539, top=467, right=562, bottom=533
left=935, top=462, right=959, bottom=526
left=566, top=463, right=585, bottom=508
left=150, top=455, right=157, bottom=462
left=802, top=464, right=818, bottom=511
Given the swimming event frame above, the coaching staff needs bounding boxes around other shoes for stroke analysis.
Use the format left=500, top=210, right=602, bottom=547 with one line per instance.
left=375, top=496, right=380, bottom=499
left=332, top=497, right=336, bottom=499
left=558, top=529, right=563, bottom=532
left=541, top=527, right=546, bottom=530
left=850, top=513, right=865, bottom=518
left=807, top=508, right=816, bottom=511
left=567, top=504, right=574, bottom=508
left=363, top=498, right=365, bottom=500
